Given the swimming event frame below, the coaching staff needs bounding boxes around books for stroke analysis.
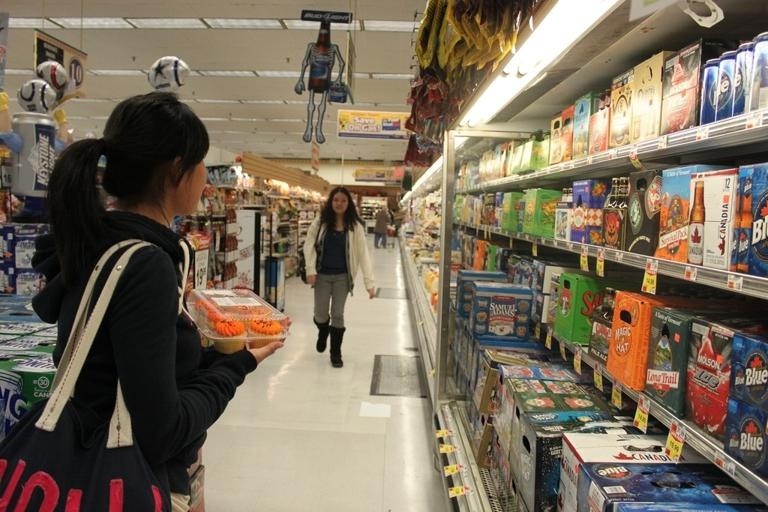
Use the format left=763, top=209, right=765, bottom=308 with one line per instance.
left=188, top=465, right=204, bottom=505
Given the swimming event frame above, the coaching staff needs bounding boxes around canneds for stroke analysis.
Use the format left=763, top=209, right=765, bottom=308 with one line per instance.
left=9, top=113, right=57, bottom=222
left=699, top=32, right=768, bottom=125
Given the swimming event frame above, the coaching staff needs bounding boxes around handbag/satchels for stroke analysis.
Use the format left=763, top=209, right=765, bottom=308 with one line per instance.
left=299, top=220, right=322, bottom=284
left=0, top=238, right=171, bottom=512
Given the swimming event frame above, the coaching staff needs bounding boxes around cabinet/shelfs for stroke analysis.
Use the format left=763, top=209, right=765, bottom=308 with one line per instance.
left=393, top=91, right=767, bottom=511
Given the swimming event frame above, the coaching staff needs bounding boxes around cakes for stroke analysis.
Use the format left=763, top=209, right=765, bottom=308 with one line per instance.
left=194, top=297, right=283, bottom=353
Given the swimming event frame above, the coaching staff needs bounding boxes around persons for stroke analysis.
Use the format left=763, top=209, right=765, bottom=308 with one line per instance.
left=374, top=207, right=391, bottom=249
left=303, top=187, right=377, bottom=368
left=30, top=92, right=284, bottom=512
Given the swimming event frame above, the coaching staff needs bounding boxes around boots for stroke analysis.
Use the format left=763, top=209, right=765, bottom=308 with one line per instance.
left=329, top=325, right=346, bottom=368
left=313, top=315, right=330, bottom=352
